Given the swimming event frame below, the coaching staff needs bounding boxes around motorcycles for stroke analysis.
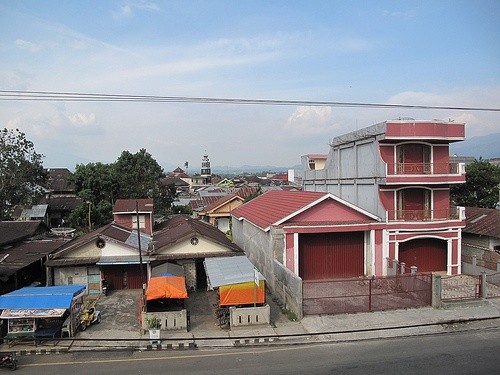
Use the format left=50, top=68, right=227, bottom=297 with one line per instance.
left=0, top=355, right=17, bottom=371
left=102, top=280, right=111, bottom=297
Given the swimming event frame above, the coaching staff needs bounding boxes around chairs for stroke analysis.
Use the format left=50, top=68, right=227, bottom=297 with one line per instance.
left=61, top=322, right=71, bottom=338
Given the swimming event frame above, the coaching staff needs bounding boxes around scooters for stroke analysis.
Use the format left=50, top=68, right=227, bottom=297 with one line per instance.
left=79, top=307, right=101, bottom=331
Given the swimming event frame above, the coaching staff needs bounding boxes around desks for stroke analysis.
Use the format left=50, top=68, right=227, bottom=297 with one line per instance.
left=33, top=327, right=60, bottom=347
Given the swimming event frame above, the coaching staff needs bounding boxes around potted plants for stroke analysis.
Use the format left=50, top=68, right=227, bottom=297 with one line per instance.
left=144, top=315, right=162, bottom=344
